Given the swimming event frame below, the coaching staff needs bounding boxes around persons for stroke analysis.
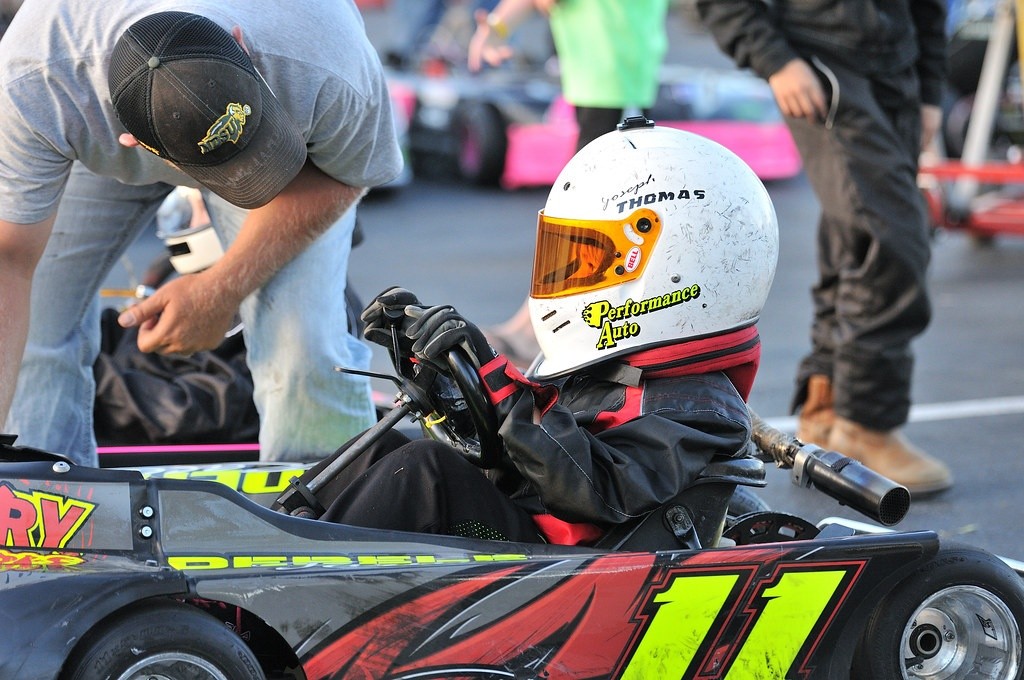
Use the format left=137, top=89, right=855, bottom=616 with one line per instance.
left=0, top=0, right=1024, bottom=494
left=272, top=114, right=779, bottom=546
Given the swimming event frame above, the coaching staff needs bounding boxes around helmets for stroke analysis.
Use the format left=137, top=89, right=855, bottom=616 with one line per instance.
left=528, top=115, right=780, bottom=381
left=155, top=186, right=224, bottom=275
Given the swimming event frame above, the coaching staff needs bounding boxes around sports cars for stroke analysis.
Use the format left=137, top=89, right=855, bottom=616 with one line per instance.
left=355, top=0, right=801, bottom=187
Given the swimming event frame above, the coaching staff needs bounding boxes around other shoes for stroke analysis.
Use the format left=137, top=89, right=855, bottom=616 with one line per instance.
left=828, top=408, right=952, bottom=498
left=796, top=374, right=836, bottom=449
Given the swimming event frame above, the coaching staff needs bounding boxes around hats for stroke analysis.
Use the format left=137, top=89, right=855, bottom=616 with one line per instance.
left=108, top=11, right=307, bottom=210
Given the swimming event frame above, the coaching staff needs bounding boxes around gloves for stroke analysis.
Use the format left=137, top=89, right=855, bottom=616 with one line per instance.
left=361, top=285, right=423, bottom=359
left=404, top=304, right=500, bottom=370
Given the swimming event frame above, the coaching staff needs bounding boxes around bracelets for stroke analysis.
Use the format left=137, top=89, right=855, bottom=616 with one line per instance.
left=486, top=14, right=511, bottom=42
